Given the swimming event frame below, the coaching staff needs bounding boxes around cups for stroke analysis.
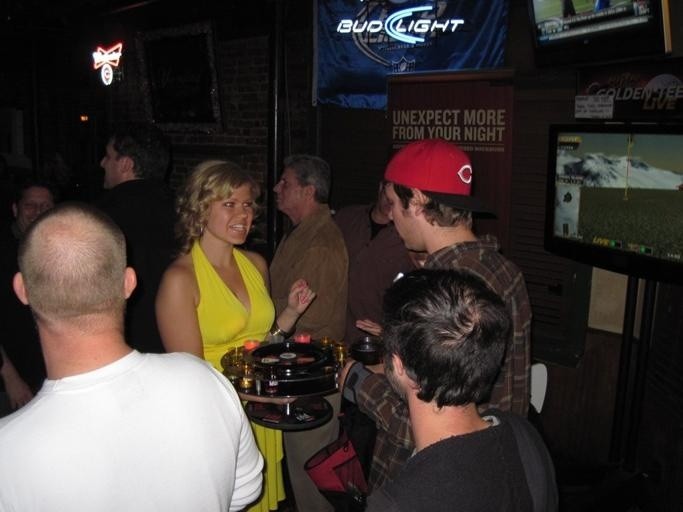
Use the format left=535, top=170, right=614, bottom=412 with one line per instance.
left=228, top=332, right=347, bottom=394
left=353, top=335, right=380, bottom=367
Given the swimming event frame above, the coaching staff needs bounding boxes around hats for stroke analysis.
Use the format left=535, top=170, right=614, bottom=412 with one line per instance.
left=384, top=138, right=492, bottom=212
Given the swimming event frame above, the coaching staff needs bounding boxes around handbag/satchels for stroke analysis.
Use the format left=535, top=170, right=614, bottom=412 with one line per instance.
left=303, top=437, right=367, bottom=512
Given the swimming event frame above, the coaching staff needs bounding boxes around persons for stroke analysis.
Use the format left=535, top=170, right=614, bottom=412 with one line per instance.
left=0, top=114, right=564, bottom=510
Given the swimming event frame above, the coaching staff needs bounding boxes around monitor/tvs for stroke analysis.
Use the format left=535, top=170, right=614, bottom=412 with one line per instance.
left=543, top=122, right=683, bottom=286
left=133, top=17, right=226, bottom=134
left=521, top=1, right=673, bottom=70
